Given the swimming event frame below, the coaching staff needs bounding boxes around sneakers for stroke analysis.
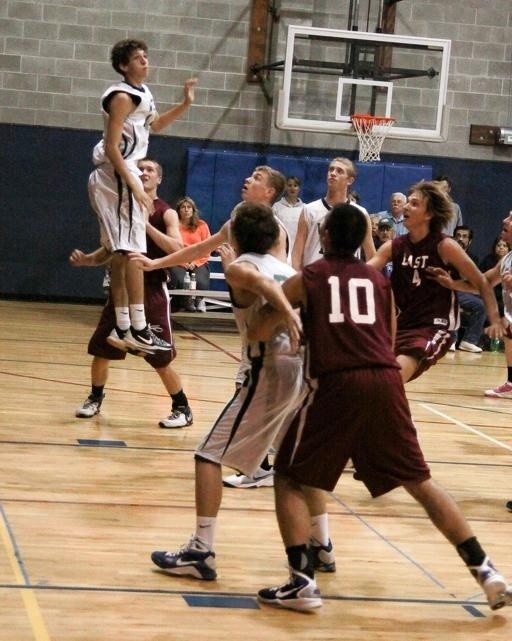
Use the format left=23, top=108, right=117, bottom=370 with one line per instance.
left=76, top=395, right=102, bottom=417
left=223, top=466, right=274, bottom=488
left=309, top=538, right=336, bottom=572
left=159, top=402, right=193, bottom=427
left=257, top=578, right=322, bottom=609
left=485, top=381, right=512, bottom=398
left=185, top=299, right=206, bottom=312
left=449, top=340, right=482, bottom=353
left=107, top=323, right=173, bottom=356
left=468, top=557, right=506, bottom=610
left=151, top=537, right=216, bottom=579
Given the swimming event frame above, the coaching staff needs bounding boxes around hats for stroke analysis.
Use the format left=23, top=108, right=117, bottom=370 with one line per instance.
left=378, top=218, right=393, bottom=227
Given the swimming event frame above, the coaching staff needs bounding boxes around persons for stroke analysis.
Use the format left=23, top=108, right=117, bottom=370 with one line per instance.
left=449, top=226, right=510, bottom=353
left=366, top=180, right=506, bottom=383
left=169, top=194, right=213, bottom=312
left=246, top=201, right=512, bottom=612
left=67, top=157, right=194, bottom=430
left=370, top=192, right=408, bottom=278
left=272, top=176, right=306, bottom=240
left=426, top=217, right=512, bottom=398
left=149, top=199, right=337, bottom=581
left=126, top=165, right=293, bottom=488
left=87, top=38, right=199, bottom=357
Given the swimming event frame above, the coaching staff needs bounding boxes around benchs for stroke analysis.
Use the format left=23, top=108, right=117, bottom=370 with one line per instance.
left=102, top=255, right=236, bottom=320
